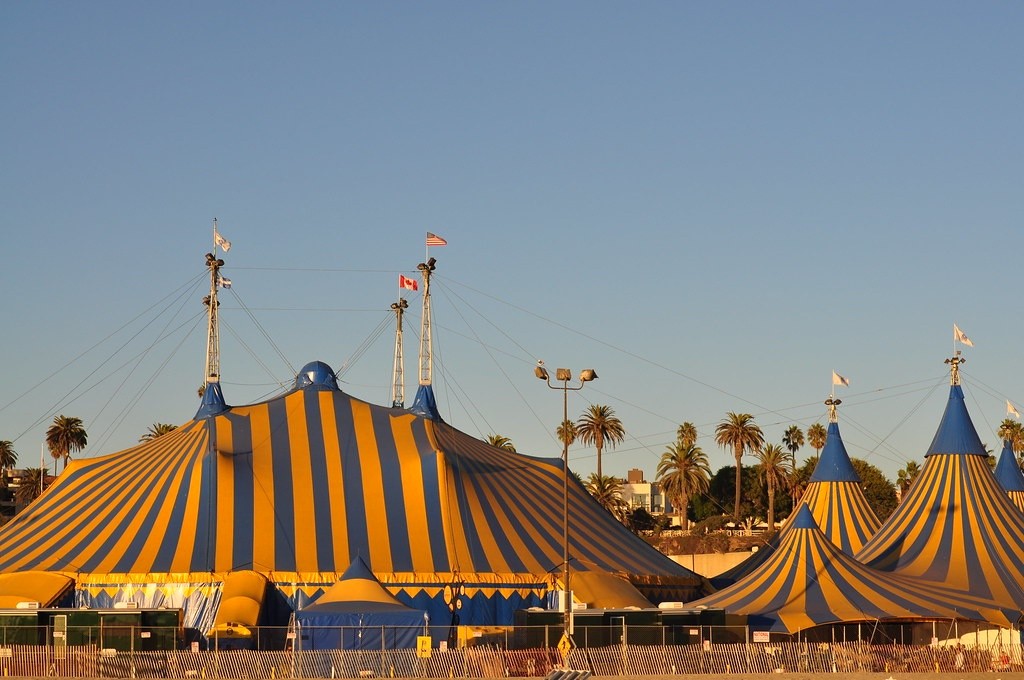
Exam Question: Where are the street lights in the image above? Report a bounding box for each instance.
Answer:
[533,361,599,680]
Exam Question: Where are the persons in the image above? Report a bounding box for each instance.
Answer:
[1,643,992,679]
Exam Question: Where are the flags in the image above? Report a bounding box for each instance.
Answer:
[1007,400,1021,419]
[832,371,850,387]
[215,233,231,253]
[427,232,447,245]
[215,276,232,289]
[954,325,975,347]
[400,275,418,292]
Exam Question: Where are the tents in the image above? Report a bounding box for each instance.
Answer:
[0,361,1024,643]
